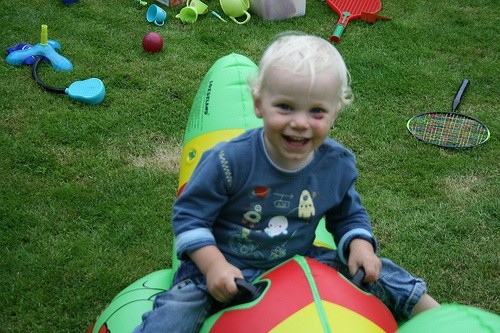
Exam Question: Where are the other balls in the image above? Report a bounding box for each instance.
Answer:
[143,31,162,51]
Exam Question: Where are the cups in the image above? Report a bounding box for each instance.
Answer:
[146,4,167,26]
[219,0,251,24]
[186,0,208,14]
[175,6,198,23]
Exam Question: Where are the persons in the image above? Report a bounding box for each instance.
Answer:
[134,36,441,333]
